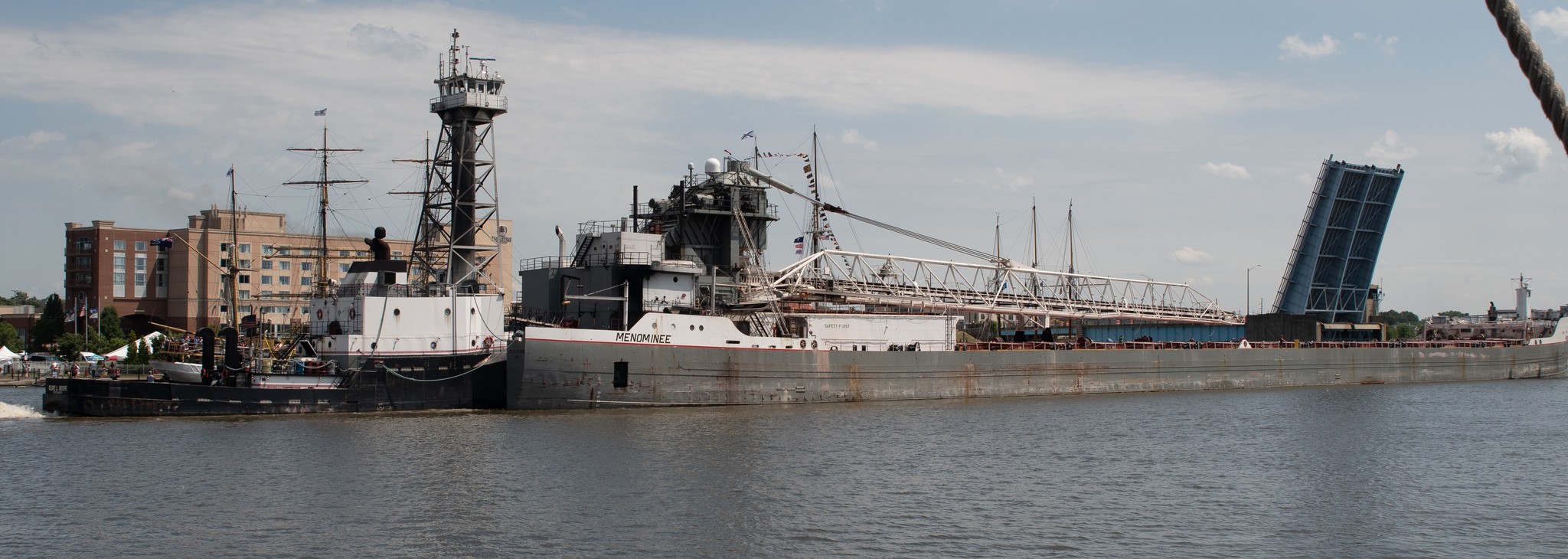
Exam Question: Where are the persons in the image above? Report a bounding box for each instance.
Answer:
[1279,334,1287,349]
[1188,336,1197,350]
[19,334,296,384]
[1118,335,1124,343]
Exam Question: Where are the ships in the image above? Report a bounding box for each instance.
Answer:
[40,31,1567,414]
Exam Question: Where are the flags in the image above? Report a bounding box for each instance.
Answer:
[89,307,98,318]
[738,129,861,282]
[64,307,75,322]
[77,298,86,317]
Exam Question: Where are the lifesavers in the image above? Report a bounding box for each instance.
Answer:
[182,343,189,351]
[317,309,323,319]
[484,335,493,346]
[222,369,228,380]
[349,308,356,319]
[200,368,206,379]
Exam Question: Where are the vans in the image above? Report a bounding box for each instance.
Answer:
[31,355,58,362]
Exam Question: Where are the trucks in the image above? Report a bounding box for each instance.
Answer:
[75,351,98,364]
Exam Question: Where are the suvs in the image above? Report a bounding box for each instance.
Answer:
[21,352,50,361]
